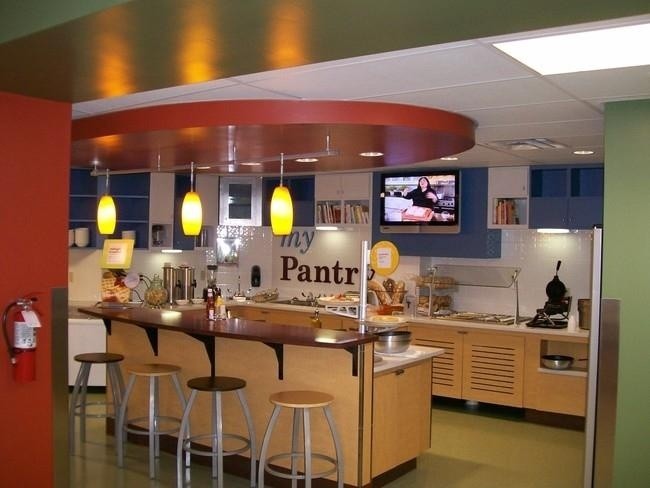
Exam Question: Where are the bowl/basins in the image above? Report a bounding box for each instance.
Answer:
[191,298,204,303]
[175,300,188,305]
[233,295,247,303]
[371,330,411,353]
[74,227,90,247]
[122,230,137,240]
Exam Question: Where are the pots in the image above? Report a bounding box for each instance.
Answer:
[541,354,587,370]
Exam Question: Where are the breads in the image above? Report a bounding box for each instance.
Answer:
[368,278,404,304]
[419,275,452,309]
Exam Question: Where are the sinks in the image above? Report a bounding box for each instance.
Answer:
[226,296,254,301]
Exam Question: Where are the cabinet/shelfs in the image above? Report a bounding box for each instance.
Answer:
[486,162,603,230]
[407,322,524,411]
[69,168,219,253]
[536,335,590,417]
[314,172,372,227]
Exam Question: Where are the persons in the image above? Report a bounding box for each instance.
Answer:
[401,176,441,210]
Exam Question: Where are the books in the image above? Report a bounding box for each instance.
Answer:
[494,198,518,223]
[316,200,369,223]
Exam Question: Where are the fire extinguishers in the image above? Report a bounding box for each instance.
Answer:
[2,291,46,383]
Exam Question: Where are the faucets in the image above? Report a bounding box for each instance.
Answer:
[237,274,241,296]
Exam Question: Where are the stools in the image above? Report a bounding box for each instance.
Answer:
[69,351,346,487]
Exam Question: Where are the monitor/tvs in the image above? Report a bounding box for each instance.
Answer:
[379,169,462,234]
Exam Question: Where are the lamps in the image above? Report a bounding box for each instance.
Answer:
[269,151,295,237]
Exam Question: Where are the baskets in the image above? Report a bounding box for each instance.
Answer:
[254,289,278,303]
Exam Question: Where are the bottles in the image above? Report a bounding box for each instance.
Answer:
[311,309,322,329]
[145,274,169,305]
[206,288,215,320]
[496,198,520,224]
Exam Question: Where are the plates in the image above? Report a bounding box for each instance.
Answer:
[318,296,360,306]
[124,302,142,307]
[363,316,408,328]
